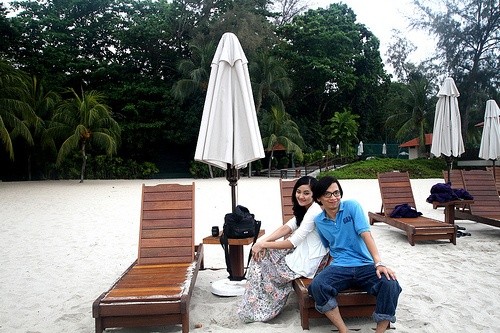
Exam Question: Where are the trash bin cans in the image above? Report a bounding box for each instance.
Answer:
[280,169,288,179]
[295,168,301,178]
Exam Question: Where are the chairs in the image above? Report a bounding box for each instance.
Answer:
[368,171,457,246]
[442,167,500,228]
[280,179,390,331]
[92,182,205,333]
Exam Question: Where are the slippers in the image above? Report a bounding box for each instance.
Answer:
[457,231,471,237]
[457,225,466,230]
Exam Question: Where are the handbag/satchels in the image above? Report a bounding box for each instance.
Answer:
[390,203,422,218]
[224,205,256,239]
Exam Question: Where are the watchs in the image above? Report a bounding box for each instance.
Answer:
[375,261,386,268]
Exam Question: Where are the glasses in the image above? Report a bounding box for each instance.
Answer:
[323,190,340,198]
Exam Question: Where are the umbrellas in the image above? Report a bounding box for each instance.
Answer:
[194,32,265,213]
[336,144,339,155]
[478,100,500,182]
[383,143,386,154]
[357,141,364,155]
[431,77,465,183]
[328,145,331,151]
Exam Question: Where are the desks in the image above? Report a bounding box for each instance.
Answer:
[426,199,474,224]
[203,230,266,277]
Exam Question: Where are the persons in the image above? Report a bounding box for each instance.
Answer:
[238,175,329,324]
[309,176,402,333]
